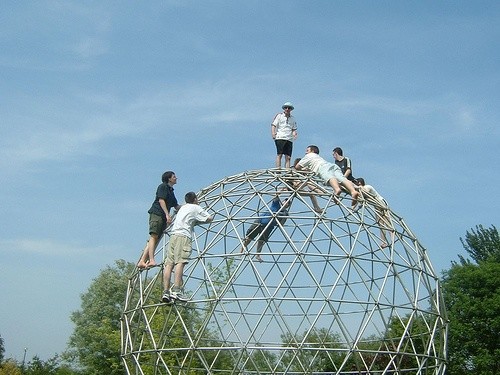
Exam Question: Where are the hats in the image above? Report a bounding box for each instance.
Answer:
[285,197,293,203]
[282,103,294,110]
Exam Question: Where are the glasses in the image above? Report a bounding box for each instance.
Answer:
[283,107,291,110]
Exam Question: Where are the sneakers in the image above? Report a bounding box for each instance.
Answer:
[163,294,174,303]
[170,290,190,301]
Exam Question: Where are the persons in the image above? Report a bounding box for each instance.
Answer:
[137,171,180,267]
[271,102,298,181]
[295,145,359,206]
[355,178,388,248]
[162,192,213,306]
[333,147,359,192]
[285,158,327,214]
[242,187,291,263]
